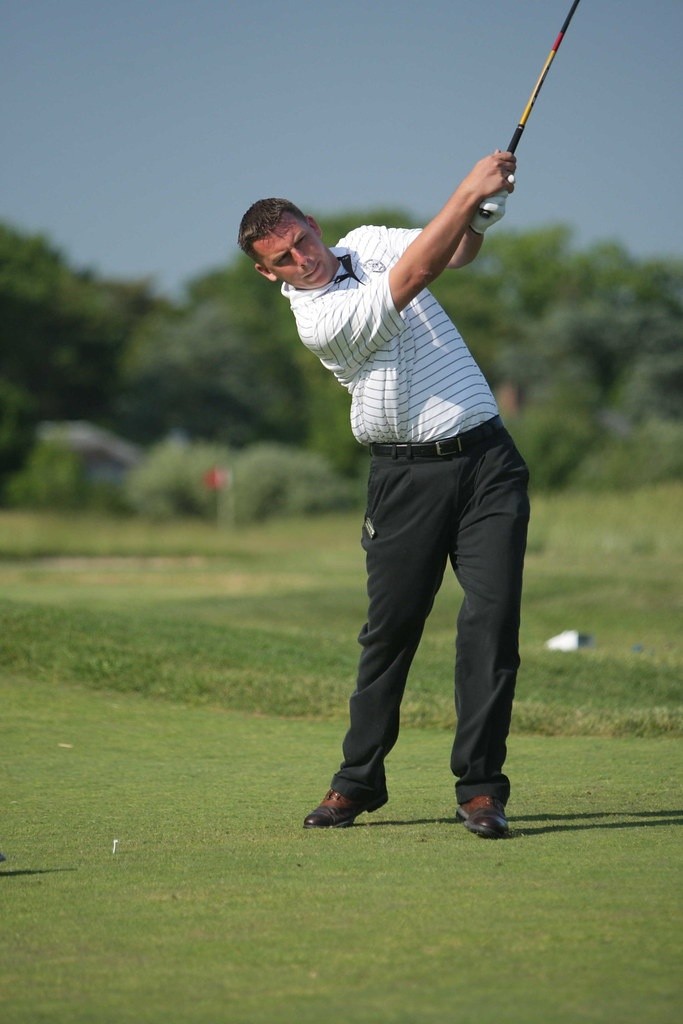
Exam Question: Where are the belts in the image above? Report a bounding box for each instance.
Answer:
[367,415,501,457]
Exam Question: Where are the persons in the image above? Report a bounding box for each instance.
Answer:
[237,148,530,839]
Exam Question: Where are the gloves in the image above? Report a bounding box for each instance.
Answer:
[468,174,517,236]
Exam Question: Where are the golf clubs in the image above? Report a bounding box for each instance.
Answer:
[479,0,580,219]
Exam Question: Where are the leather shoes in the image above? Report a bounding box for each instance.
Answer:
[304,789,389,829]
[456,795,510,839]
